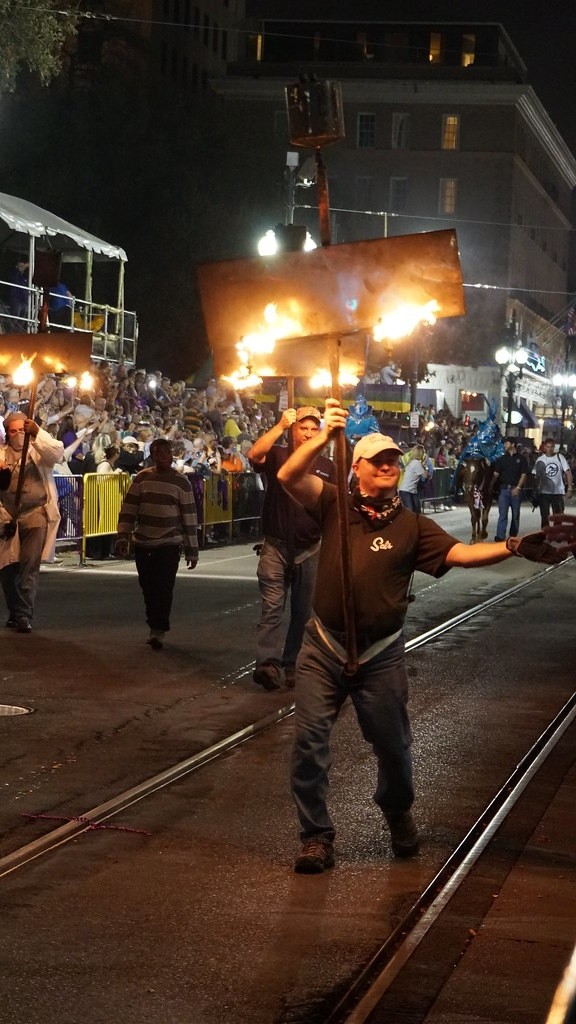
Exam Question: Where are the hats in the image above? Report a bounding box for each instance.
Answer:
[352,432,404,464]
[2,412,28,430]
[501,436,517,445]
[295,407,321,425]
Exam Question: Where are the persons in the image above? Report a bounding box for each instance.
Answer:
[116,438,198,650]
[532,438,573,531]
[487,437,528,542]
[248,406,333,689]
[0,412,64,632]
[0,360,576,559]
[398,444,428,515]
[277,398,569,874]
[6,260,73,334]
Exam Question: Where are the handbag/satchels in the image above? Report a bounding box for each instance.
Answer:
[531,490,539,509]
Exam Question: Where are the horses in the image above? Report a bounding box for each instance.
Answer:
[461,459,494,544]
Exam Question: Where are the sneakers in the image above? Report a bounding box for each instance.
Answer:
[383,811,420,860]
[285,664,297,688]
[255,663,281,691]
[294,840,336,874]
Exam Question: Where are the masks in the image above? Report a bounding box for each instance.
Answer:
[9,432,32,452]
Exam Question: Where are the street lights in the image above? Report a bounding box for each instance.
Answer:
[552,372,576,455]
[493,344,530,437]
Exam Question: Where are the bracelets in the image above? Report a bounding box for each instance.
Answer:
[517,487,521,489]
[568,486,573,491]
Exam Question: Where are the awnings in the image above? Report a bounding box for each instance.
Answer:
[520,399,539,428]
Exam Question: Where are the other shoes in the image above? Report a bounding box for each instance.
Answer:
[6,612,17,628]
[88,522,260,560]
[17,617,32,633]
[145,630,164,652]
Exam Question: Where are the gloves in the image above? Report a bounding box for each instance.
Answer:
[506,532,569,565]
